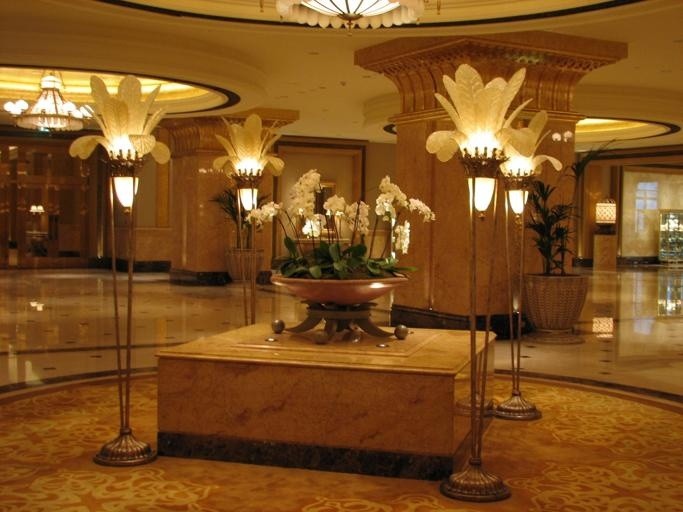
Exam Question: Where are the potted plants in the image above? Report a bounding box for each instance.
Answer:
[209,188,272,290]
[517,138,623,345]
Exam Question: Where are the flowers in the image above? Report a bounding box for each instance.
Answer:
[243,168,436,278]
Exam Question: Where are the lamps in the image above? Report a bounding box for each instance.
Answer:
[4,68,95,133]
[275,0,426,37]
[593,198,617,235]
[69,73,170,467]
[424,62,535,501]
[28,298,45,312]
[592,309,615,340]
[28,204,45,215]
[494,105,563,421]
[211,113,284,331]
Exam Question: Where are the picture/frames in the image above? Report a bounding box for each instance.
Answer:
[312,180,339,235]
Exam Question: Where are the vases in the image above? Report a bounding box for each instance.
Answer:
[267,275,410,344]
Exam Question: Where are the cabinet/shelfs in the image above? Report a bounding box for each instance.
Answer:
[657,209,683,266]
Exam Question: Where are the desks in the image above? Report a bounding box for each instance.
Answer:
[153,322,498,480]
